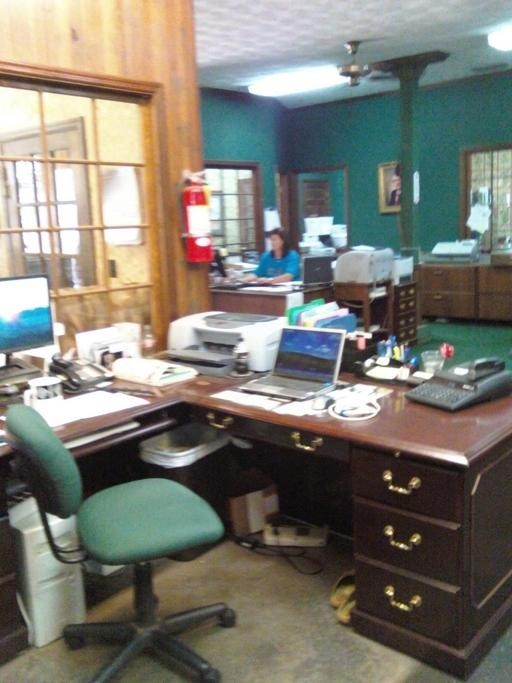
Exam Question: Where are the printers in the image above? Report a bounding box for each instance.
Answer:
[333,245,395,284]
[166,310,290,373]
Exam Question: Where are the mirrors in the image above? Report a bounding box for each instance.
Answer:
[290,165,349,254]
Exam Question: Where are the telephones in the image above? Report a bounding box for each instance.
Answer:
[49,353,114,392]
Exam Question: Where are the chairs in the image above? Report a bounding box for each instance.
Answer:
[1,403,237,682]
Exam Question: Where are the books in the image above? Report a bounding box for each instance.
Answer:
[112,356,198,387]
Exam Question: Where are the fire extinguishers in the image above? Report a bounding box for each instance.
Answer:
[180,171,215,263]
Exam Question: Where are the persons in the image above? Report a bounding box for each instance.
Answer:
[388,175,402,205]
[243,228,300,283]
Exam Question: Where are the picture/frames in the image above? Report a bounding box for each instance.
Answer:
[377,160,402,214]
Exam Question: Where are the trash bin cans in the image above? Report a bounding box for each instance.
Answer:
[136,424,233,561]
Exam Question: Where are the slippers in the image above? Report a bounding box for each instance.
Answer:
[335,589,357,624]
[329,569,358,608]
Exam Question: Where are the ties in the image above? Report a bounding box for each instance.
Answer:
[395,191,400,204]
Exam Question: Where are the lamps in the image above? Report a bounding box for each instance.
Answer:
[338,41,371,87]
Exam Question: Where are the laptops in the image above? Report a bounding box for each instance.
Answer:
[291,255,336,287]
[239,325,347,401]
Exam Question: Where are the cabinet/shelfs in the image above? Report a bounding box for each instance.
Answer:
[391,280,420,349]
[419,261,477,319]
[335,276,391,339]
[477,265,511,320]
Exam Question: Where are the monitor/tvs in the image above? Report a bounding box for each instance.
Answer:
[1,274,56,381]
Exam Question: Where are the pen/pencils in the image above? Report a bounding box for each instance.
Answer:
[393,345,405,361]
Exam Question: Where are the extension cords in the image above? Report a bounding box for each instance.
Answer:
[260,523,330,548]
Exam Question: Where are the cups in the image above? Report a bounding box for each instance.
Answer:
[422,350,445,374]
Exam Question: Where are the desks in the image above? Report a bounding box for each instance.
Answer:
[0,345,512,682]
[210,275,333,314]
